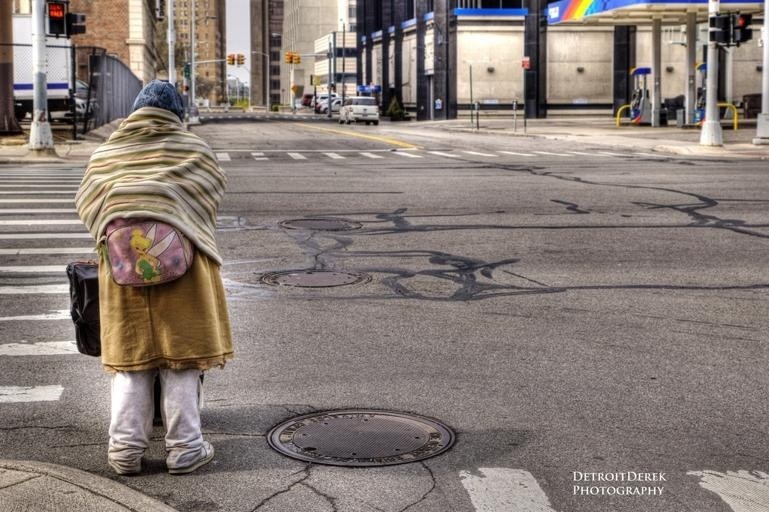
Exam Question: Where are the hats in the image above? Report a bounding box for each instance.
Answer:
[132,80,184,120]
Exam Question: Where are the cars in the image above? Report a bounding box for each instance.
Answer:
[301,93,341,114]
[338,96,378,125]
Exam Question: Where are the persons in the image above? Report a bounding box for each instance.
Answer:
[75,80,235,474]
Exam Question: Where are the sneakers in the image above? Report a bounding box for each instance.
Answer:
[108,456,142,476]
[168,439,215,476]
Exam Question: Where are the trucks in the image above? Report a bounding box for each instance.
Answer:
[11,13,72,122]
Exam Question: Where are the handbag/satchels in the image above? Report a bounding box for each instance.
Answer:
[66,261,101,357]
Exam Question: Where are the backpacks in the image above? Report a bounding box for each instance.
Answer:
[105,218,196,288]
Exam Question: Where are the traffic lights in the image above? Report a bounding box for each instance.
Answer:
[285,51,300,64]
[227,54,234,65]
[44,0,85,39]
[709,12,752,42]
[236,54,244,65]
